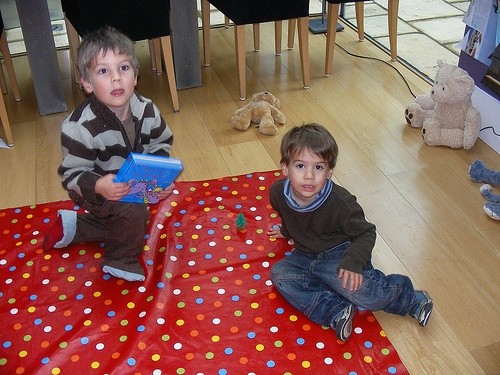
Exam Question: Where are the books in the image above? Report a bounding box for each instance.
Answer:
[109,152,183,203]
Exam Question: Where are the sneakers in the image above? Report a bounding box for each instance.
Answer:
[334,303,356,341]
[408,290,434,327]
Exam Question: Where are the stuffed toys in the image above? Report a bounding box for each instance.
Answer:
[405,61,483,150]
[467,159,500,221]
[231,91,286,136]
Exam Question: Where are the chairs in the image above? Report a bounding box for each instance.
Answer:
[61,0,179,117]
[201,0,311,100]
[323,0,401,78]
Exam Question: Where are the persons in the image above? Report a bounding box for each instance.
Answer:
[266,122,433,341]
[42,29,176,281]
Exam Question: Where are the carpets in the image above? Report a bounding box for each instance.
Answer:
[0,168,410,375]
